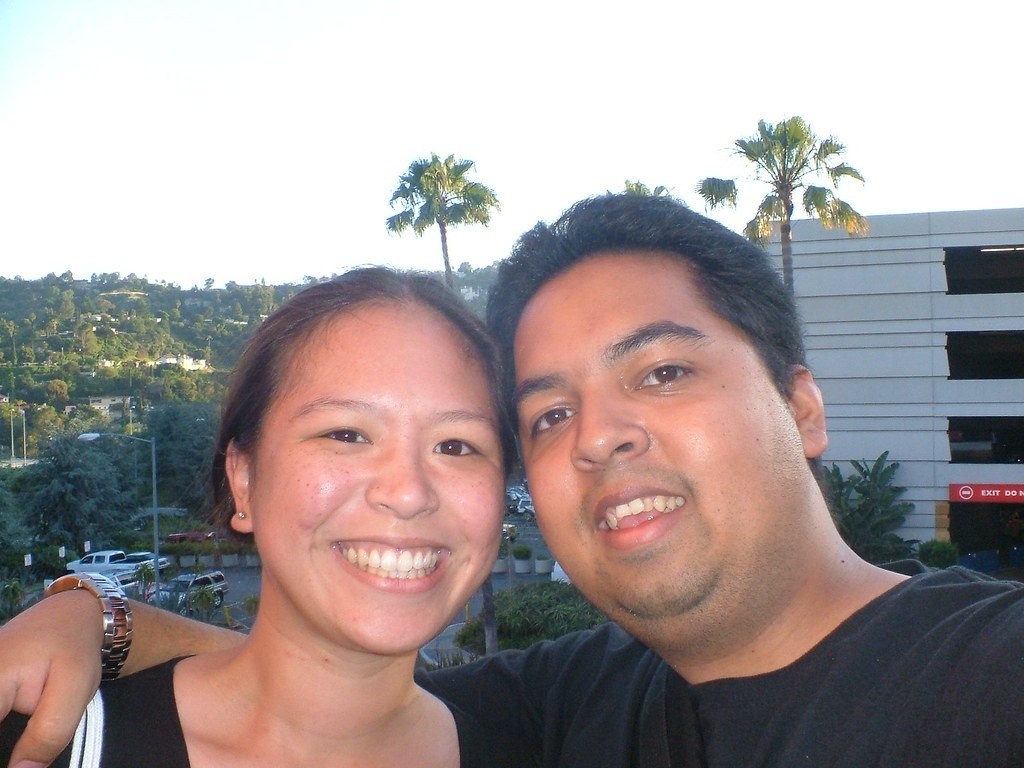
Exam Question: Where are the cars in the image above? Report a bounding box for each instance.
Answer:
[65,547,173,605]
[505,486,537,524]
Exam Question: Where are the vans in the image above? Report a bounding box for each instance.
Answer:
[162,533,221,548]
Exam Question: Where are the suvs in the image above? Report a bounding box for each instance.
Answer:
[147,570,230,618]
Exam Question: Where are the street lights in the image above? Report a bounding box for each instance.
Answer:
[77,431,162,608]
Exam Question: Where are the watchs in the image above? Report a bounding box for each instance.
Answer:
[42,572,134,686]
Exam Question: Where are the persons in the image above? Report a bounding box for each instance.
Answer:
[1,191,1023,768]
[1,263,548,768]
